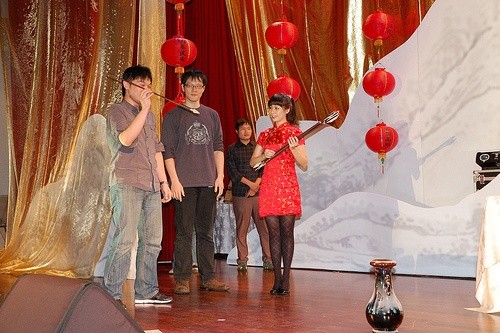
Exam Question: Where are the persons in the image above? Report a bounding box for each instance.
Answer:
[226,120,274,271]
[160,70,230,295]
[106,66,173,309]
[249,93,308,294]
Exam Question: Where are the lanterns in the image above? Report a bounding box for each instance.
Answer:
[361,9,395,60]
[161,35,198,83]
[267,74,301,101]
[365,122,399,174]
[265,19,299,71]
[162,97,186,118]
[165,0,191,19]
[362,68,395,117]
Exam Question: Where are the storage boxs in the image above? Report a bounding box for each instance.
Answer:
[472,170,500,193]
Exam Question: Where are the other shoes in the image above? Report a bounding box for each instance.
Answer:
[264,262,274,269]
[176,280,190,293]
[200,279,230,290]
[238,263,247,271]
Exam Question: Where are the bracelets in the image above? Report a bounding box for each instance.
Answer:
[160,181,168,187]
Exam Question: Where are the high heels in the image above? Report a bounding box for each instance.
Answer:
[270,288,280,295]
[278,288,289,294]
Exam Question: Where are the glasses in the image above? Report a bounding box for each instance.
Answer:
[185,83,204,89]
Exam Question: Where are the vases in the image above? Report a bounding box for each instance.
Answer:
[365,259,404,333]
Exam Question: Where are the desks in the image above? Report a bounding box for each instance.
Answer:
[463,196,500,314]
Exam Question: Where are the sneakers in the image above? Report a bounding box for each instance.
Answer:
[135,293,172,303]
[116,299,126,309]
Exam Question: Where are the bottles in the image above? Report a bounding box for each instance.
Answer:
[365,259,405,333]
